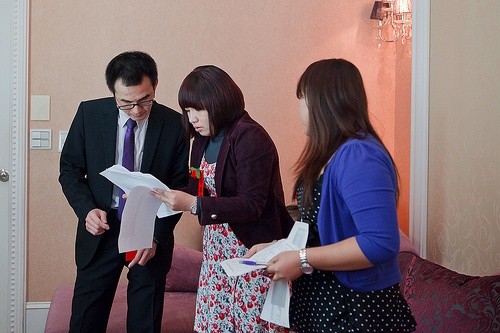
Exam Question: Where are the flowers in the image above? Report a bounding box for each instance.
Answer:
[189,166,203,181]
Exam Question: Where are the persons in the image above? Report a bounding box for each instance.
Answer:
[121,64,295,333]
[57,54,193,333]
[241,58,417,333]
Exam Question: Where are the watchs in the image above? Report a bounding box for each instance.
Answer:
[299,249,314,274]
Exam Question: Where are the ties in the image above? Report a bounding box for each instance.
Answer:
[118,118,137,221]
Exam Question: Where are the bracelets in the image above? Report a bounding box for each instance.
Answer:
[190,196,198,214]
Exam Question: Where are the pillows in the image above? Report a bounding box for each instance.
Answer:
[163,243,203,292]
[400,256,500,333]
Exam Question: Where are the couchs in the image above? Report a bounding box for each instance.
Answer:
[44,204,500,333]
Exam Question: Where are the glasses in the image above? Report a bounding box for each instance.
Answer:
[114,87,156,110]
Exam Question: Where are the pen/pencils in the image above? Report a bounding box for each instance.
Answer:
[240,260,276,265]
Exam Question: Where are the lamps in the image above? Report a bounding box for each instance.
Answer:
[368,0,412,48]
